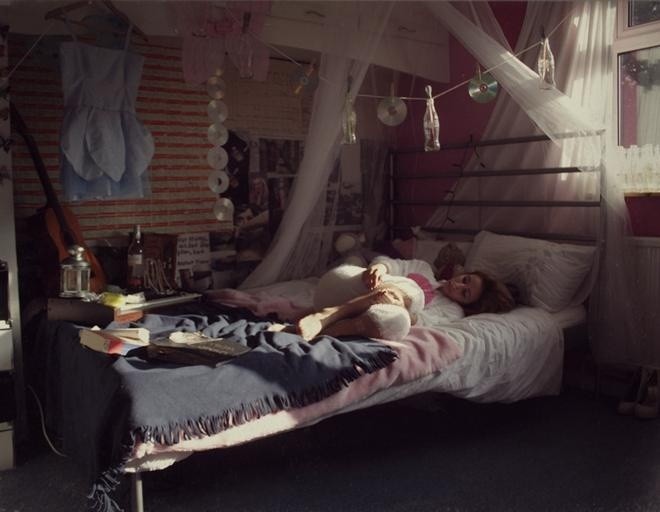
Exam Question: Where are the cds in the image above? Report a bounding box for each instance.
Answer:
[207,170,229,194]
[468,72,498,103]
[376,97,408,126]
[213,198,235,220]
[206,77,225,99]
[208,124,229,146]
[207,146,228,169]
[207,100,229,123]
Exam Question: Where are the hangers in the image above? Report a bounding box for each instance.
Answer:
[44,1,150,45]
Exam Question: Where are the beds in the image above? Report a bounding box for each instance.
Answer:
[26,129,608,510]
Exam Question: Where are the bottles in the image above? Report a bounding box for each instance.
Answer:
[613,143,660,195]
[127,225,145,295]
[238,28,253,80]
[537,36,556,91]
[423,97,441,153]
[341,92,360,146]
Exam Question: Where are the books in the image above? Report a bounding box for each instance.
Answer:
[73,326,252,367]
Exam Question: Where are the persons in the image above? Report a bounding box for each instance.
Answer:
[263,254,524,347]
[365,182,392,229]
[222,139,305,241]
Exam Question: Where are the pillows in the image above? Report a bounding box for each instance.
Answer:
[463,230,600,314]
